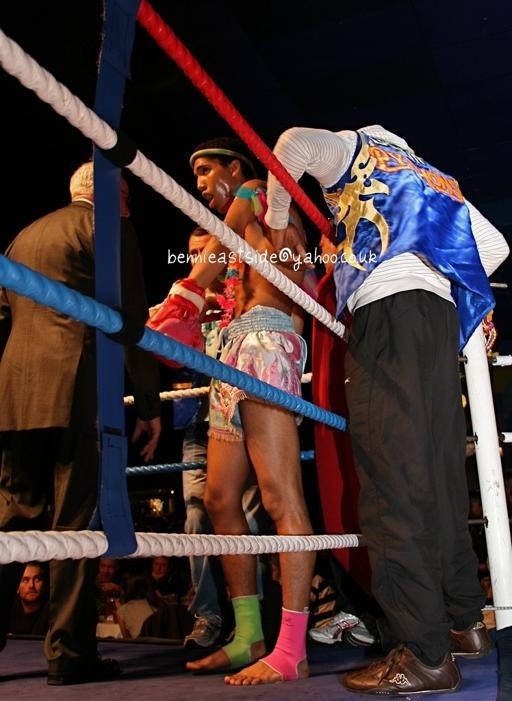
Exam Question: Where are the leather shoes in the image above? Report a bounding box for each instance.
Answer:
[48,658,121,685]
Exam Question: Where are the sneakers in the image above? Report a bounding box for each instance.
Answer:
[184,615,221,648]
[309,611,491,696]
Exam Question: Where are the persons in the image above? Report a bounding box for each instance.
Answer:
[264,122,511,698]
[309,232,401,649]
[143,134,316,687]
[2,481,512,648]
[0,154,149,688]
[171,225,277,650]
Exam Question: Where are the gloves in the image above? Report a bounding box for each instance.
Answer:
[146,278,205,368]
